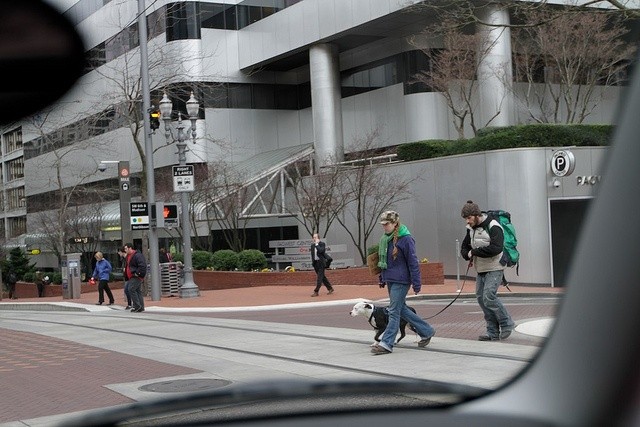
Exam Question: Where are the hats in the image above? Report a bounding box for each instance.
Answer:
[460,200,481,217]
[379,210,399,225]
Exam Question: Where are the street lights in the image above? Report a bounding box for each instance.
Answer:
[160,90,199,299]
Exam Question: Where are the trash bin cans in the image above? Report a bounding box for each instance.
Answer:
[146,263,182,298]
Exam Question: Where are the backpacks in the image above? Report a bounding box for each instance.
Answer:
[467,209,520,292]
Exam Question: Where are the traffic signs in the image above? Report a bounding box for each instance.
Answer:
[172,166,194,193]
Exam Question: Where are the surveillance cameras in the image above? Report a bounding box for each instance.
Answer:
[97,159,108,172]
[553,177,560,188]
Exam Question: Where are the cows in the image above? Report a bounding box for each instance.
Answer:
[349,296,425,349]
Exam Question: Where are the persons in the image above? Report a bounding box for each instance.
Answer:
[461,200,520,341]
[91,251,114,305]
[117,246,132,310]
[370,210,436,355]
[124,243,147,312]
[310,233,335,297]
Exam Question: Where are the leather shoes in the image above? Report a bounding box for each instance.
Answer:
[126,305,131,309]
[96,301,102,305]
[326,288,334,295]
[312,292,318,297]
[138,308,144,312]
[417,328,437,347]
[107,303,114,305]
[479,335,499,341]
[131,308,136,312]
[501,322,517,339]
[372,345,391,354]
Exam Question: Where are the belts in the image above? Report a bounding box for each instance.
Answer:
[314,260,320,262]
